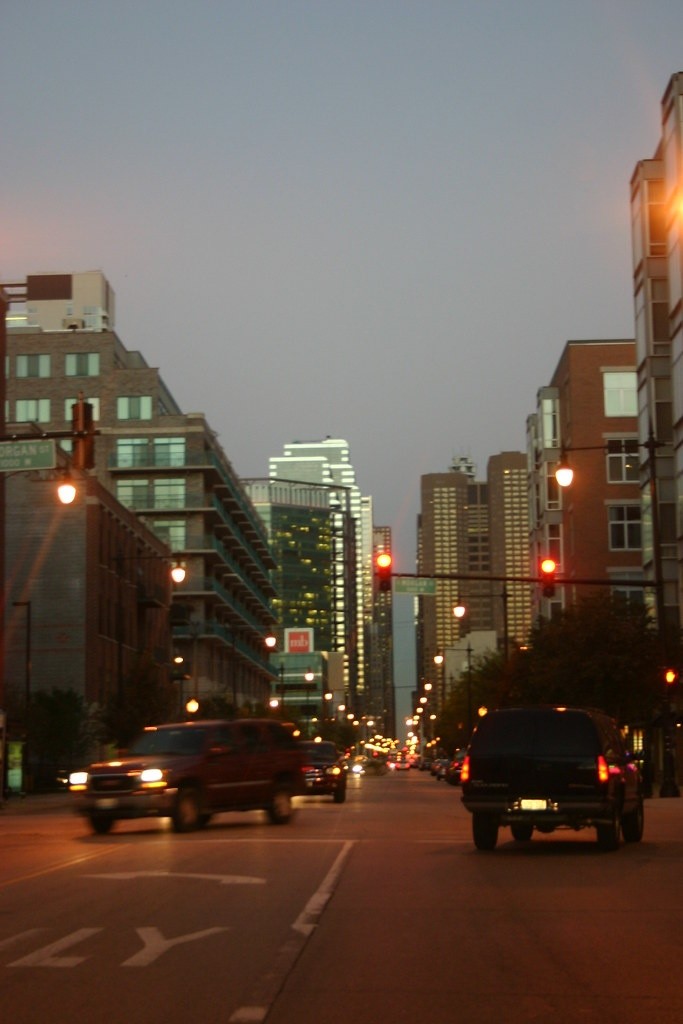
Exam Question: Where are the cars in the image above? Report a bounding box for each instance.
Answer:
[341,741,463,787]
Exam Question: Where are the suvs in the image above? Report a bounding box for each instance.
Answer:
[457,703,647,849]
[296,737,348,804]
[68,717,308,833]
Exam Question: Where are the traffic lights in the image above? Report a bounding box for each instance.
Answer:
[536,555,556,597]
[373,552,393,594]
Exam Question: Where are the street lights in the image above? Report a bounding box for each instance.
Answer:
[108,550,192,711]
[453,589,510,664]
[427,645,476,722]
[280,662,316,715]
[553,435,670,665]
